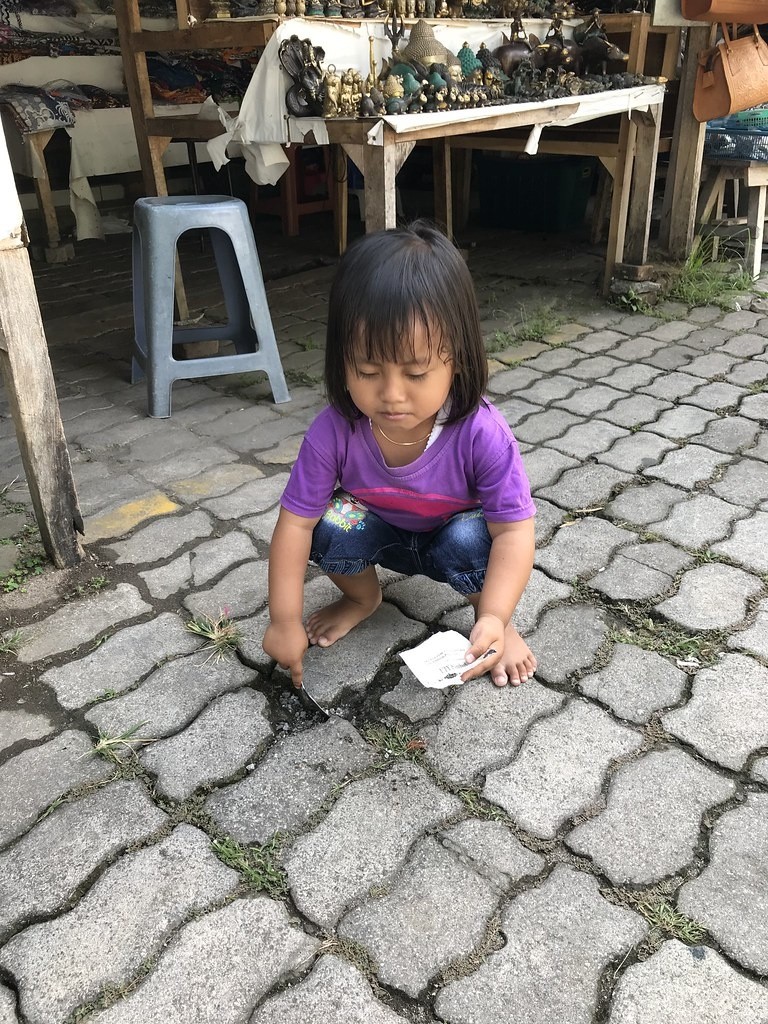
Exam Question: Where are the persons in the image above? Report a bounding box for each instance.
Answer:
[259,219,535,689]
[272,0,644,118]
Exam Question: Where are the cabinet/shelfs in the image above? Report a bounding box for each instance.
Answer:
[114,0,666,297]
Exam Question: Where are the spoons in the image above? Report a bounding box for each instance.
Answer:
[301,681,331,718]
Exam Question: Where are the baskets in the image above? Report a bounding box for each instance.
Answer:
[738,109,768,129]
[703,129,768,160]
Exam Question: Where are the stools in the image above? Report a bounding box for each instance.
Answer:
[249,143,347,256]
[129,196,290,420]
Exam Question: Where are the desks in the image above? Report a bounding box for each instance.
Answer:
[0,12,239,264]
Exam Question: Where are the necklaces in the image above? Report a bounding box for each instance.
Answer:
[378,424,430,446]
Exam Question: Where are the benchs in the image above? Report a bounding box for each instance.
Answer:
[691,158,768,282]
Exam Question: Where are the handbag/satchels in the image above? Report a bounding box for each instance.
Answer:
[692,21,768,123]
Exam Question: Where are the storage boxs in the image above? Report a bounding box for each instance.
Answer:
[473,153,601,231]
